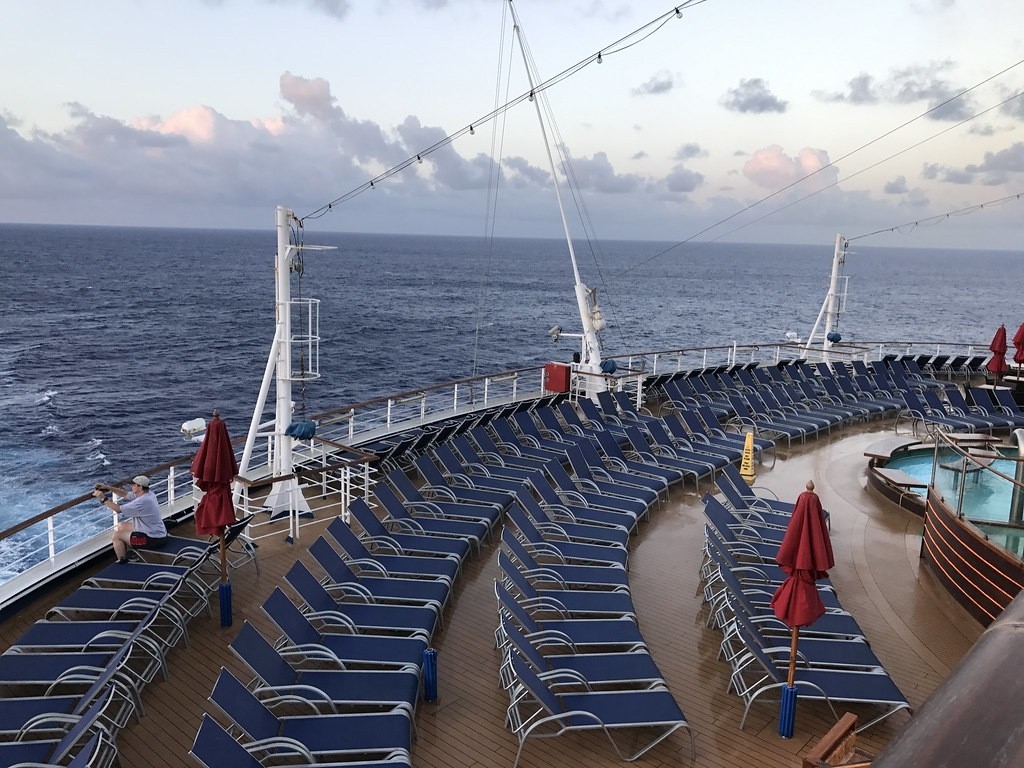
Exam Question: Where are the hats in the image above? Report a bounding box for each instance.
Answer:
[128,475,151,489]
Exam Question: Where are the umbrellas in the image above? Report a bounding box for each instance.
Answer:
[1012,322,1024,381]
[986,324,1008,390]
[192,409,239,583]
[770,479,835,688]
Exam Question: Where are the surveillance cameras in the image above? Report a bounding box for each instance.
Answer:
[549,325,559,334]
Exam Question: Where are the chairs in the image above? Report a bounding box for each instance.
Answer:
[0,354,1024,768]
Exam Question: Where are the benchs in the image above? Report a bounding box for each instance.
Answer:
[864,434,922,458]
[946,433,1003,446]
[940,448,996,490]
[872,466,927,491]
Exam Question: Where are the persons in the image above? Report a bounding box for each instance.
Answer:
[92,476,168,563]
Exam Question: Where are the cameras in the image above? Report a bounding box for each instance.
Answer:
[94,486,102,491]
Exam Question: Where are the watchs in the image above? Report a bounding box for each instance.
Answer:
[101,497,108,503]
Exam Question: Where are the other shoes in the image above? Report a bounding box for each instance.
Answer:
[127,551,139,561]
